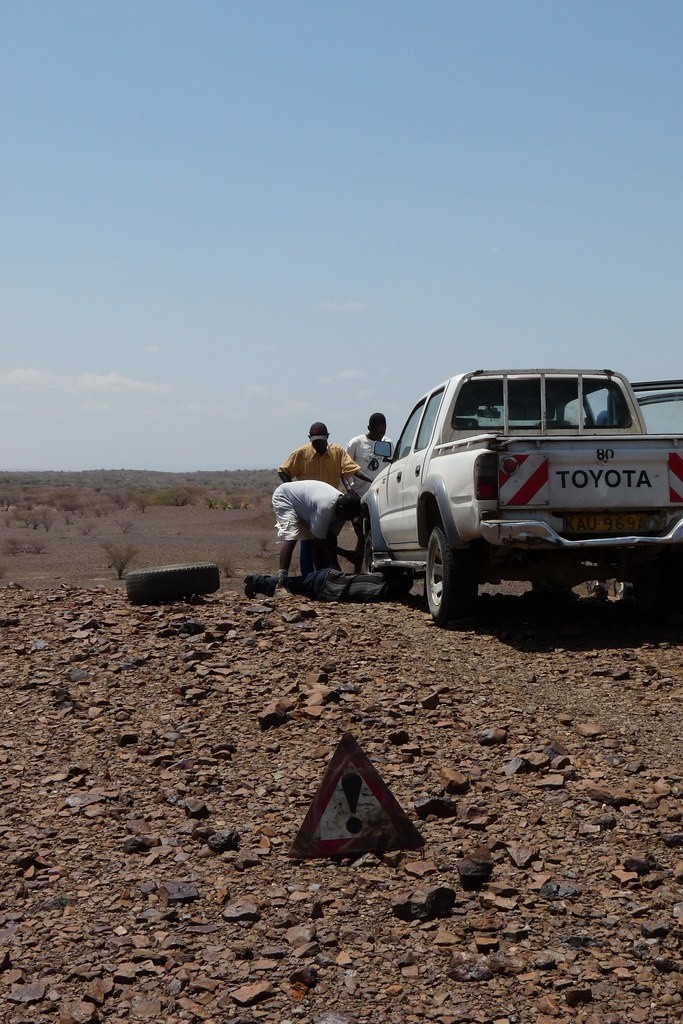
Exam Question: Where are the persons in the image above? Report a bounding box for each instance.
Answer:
[345,412,394,574]
[272,480,361,597]
[278,421,374,572]
[243,546,423,599]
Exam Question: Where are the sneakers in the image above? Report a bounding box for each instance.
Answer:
[244,573,256,599]
[273,583,293,602]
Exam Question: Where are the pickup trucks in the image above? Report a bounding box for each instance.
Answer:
[350,368,682,628]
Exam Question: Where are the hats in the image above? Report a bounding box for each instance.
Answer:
[308,421,327,443]
